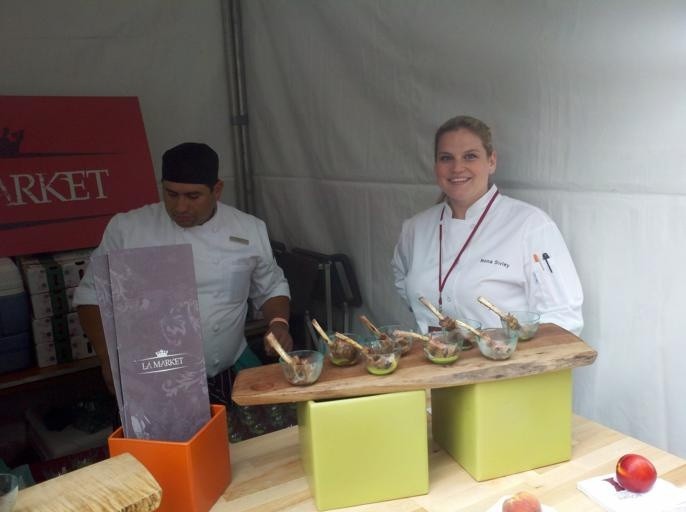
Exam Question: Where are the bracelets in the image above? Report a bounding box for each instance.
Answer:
[269,317,289,327]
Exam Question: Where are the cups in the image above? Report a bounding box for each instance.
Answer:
[442,319,481,351]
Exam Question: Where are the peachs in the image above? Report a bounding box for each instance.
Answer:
[502,490,542,512]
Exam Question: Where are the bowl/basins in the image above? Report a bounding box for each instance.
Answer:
[279,350,324,387]
[500,311,540,342]
[422,331,464,365]
[374,326,413,358]
[475,328,518,360]
[359,340,402,376]
[319,331,364,368]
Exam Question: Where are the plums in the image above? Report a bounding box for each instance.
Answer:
[615,454,657,494]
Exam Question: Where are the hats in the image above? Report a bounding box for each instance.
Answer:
[162,142,220,186]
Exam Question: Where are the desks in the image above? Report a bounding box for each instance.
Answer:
[207,413,686,510]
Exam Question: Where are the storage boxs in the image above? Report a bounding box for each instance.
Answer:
[108,403,232,511]
[296,389,429,512]
[431,369,573,480]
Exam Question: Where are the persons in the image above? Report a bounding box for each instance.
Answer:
[72,142,293,407]
[391,115,584,338]
